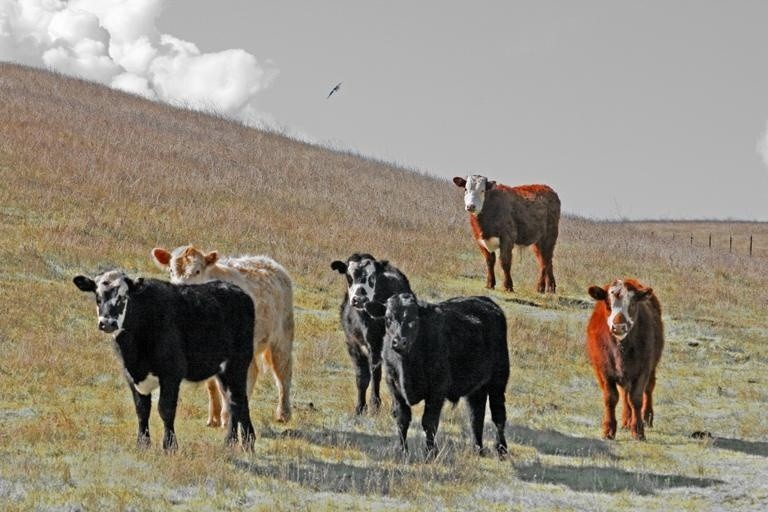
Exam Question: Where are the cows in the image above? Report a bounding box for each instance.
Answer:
[363,293,510,463]
[73,269,255,454]
[586,276,665,443]
[453,175,561,293]
[151,246,294,427]
[330,253,417,417]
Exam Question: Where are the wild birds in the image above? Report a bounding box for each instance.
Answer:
[328,82,342,97]
